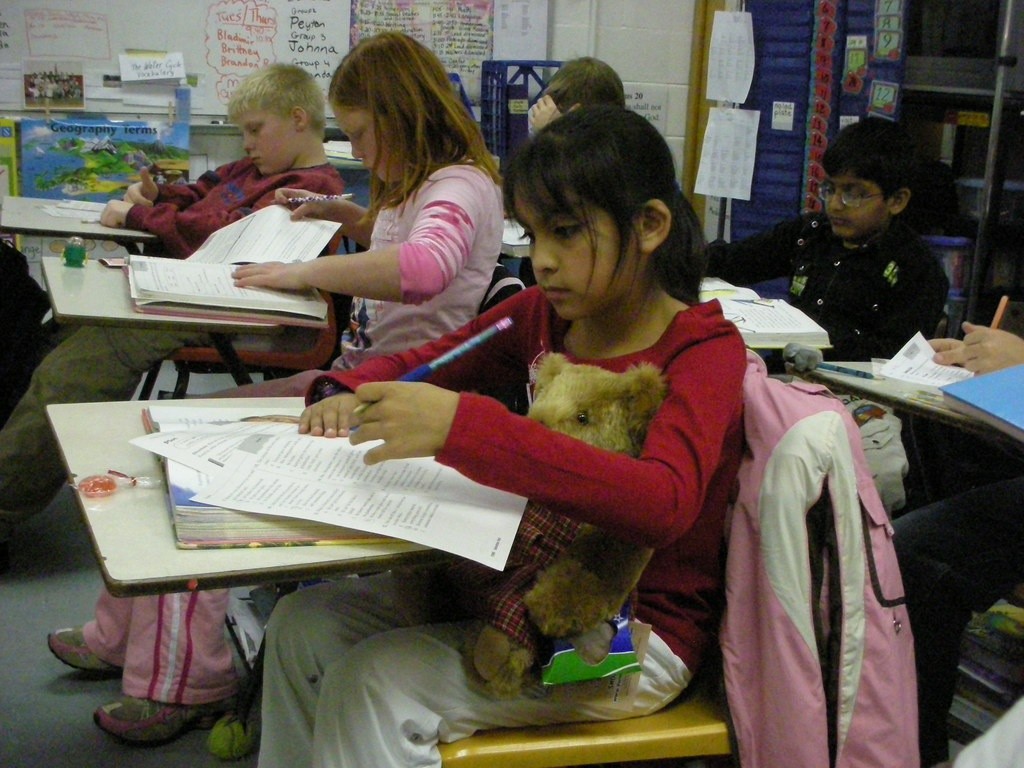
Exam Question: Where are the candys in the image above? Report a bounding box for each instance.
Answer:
[79,476,116,497]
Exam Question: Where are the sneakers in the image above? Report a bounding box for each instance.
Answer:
[48,626,122,672]
[94,693,223,742]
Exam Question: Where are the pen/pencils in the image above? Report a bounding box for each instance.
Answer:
[353,317,515,413]
[990,295,1010,328]
[272,193,354,206]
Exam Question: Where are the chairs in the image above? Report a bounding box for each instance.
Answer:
[437,348,920,768]
[140,224,341,400]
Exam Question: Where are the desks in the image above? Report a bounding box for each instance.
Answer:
[786,361,1013,445]
[42,256,286,387]
[44,397,448,599]
[0,196,157,255]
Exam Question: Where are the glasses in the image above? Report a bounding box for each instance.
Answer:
[818,180,884,209]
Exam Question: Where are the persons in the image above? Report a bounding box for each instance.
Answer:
[249,107,746,768]
[682,117,949,395]
[514,56,625,140]
[39,33,504,745]
[0,61,341,578]
[859,319,1024,768]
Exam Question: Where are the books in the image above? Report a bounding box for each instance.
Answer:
[879,326,1024,444]
[496,212,532,245]
[318,127,364,164]
[51,188,103,222]
[126,203,342,320]
[699,262,829,350]
[693,106,766,205]
[686,8,763,101]
[140,392,531,572]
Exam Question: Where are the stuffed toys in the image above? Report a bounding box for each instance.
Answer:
[463,341,666,701]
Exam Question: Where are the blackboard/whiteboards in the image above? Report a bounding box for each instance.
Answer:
[0,0,557,124]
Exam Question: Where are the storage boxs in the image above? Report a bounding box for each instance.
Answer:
[482,60,562,172]
[958,178,1024,231]
[921,235,1018,305]
[0,87,191,254]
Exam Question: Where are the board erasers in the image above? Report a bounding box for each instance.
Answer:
[210,119,220,125]
